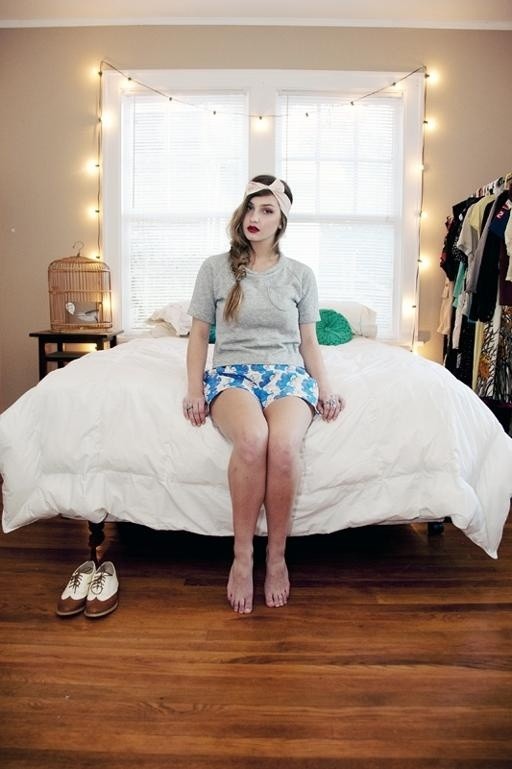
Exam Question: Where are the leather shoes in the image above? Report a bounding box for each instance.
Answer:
[55,561,119,617]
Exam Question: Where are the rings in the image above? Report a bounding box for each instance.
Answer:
[186,404,192,412]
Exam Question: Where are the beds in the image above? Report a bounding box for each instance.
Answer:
[0,329,512,568]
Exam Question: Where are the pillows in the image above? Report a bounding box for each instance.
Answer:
[145,298,379,340]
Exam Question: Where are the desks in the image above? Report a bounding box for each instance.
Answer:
[29,329,125,381]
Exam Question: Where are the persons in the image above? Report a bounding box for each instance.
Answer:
[181,174,345,614]
